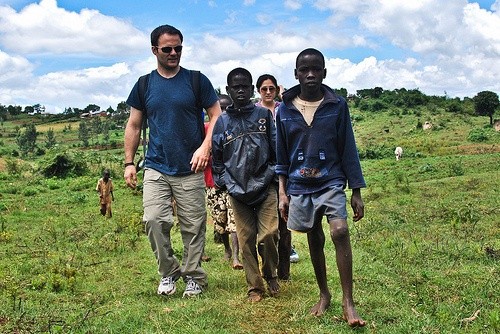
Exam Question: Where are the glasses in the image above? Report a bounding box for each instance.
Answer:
[155,45,182,53]
[259,86,277,93]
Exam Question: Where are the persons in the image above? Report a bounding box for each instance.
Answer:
[96,169,114,218]
[202,94,244,270]
[124,24,223,300]
[250,74,300,279]
[211,68,281,304]
[276,47,367,328]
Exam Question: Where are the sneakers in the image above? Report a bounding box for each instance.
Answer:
[157,273,180,296]
[290,245,300,262]
[182,275,202,298]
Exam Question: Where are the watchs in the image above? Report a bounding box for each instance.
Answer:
[124,162,135,168]
